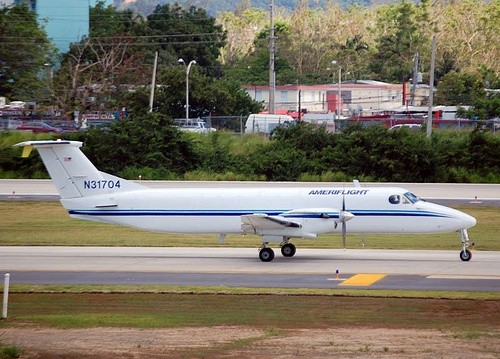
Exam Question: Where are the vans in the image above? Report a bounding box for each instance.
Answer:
[387,124,423,131]
[245,114,297,134]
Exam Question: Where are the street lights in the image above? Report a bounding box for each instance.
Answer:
[44,63,56,107]
[177,58,197,123]
[326,60,345,122]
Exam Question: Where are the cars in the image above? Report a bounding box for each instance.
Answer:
[16,122,63,133]
[177,122,216,134]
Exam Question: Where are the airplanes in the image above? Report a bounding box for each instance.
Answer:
[13,139,479,263]
